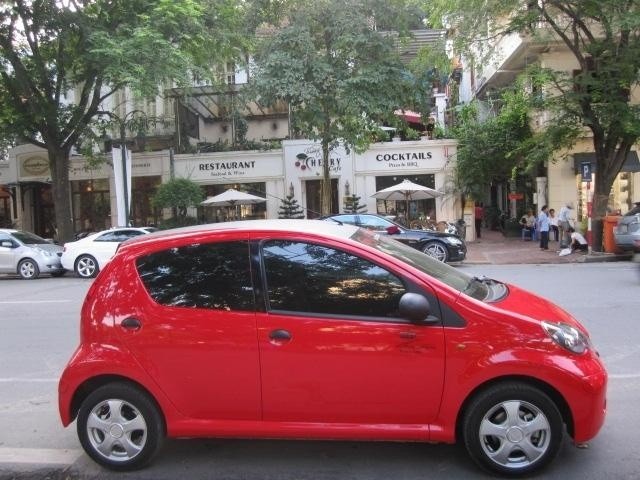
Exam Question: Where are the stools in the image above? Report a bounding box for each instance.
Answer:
[522,227,537,242]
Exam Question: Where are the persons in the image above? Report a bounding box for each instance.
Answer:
[547,209,559,242]
[557,201,576,245]
[475,200,484,238]
[537,203,550,252]
[518,207,536,234]
[565,227,588,252]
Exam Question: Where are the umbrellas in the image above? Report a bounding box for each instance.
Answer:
[369,178,447,228]
[199,187,270,220]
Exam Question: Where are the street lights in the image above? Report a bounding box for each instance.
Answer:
[97,110,148,229]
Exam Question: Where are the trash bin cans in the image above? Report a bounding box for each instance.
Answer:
[602,216,624,254]
[484,206,499,231]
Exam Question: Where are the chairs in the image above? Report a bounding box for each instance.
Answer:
[410,218,449,233]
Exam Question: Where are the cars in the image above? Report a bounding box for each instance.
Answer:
[0,227,166,279]
[318,214,466,263]
[59,219,607,480]
[613,201,640,251]
